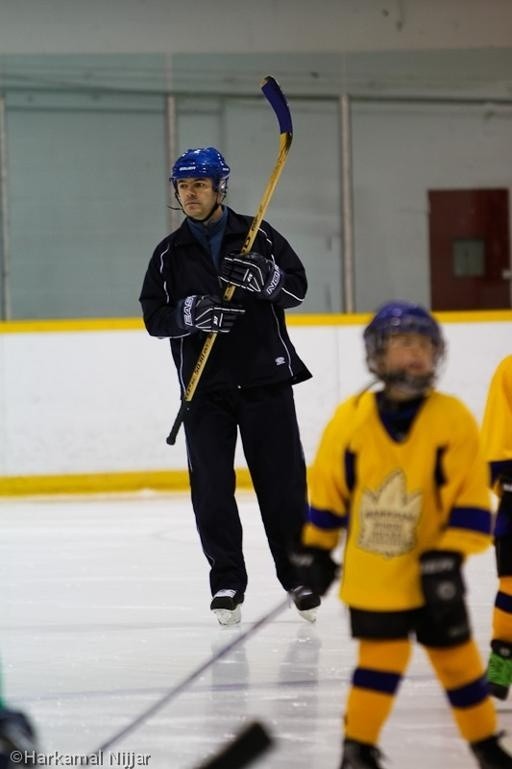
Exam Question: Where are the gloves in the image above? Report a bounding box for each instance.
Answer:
[216,251,286,300]
[419,549,465,607]
[183,294,246,333]
[289,546,341,594]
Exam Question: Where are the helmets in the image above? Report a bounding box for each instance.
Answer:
[170,146,230,191]
[362,302,445,393]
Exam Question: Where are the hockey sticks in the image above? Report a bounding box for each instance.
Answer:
[166,74,291,443]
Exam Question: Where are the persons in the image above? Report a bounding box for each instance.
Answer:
[295,300,512,769]
[139,145,322,612]
[479,354,512,700]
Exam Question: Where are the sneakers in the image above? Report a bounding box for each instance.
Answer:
[292,585,321,610]
[210,588,244,610]
[486,640,512,699]
[341,738,382,769]
[470,734,512,769]
